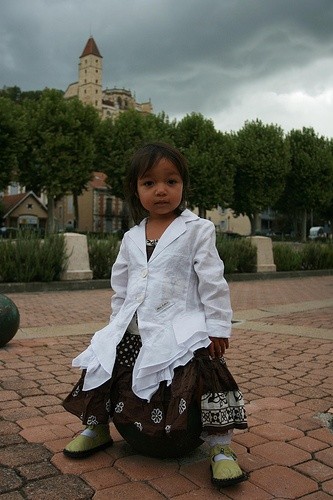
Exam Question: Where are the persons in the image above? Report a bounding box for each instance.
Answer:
[62,141,243,486]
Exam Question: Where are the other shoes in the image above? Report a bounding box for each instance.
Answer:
[63,425,113,458]
[208,445,245,487]
